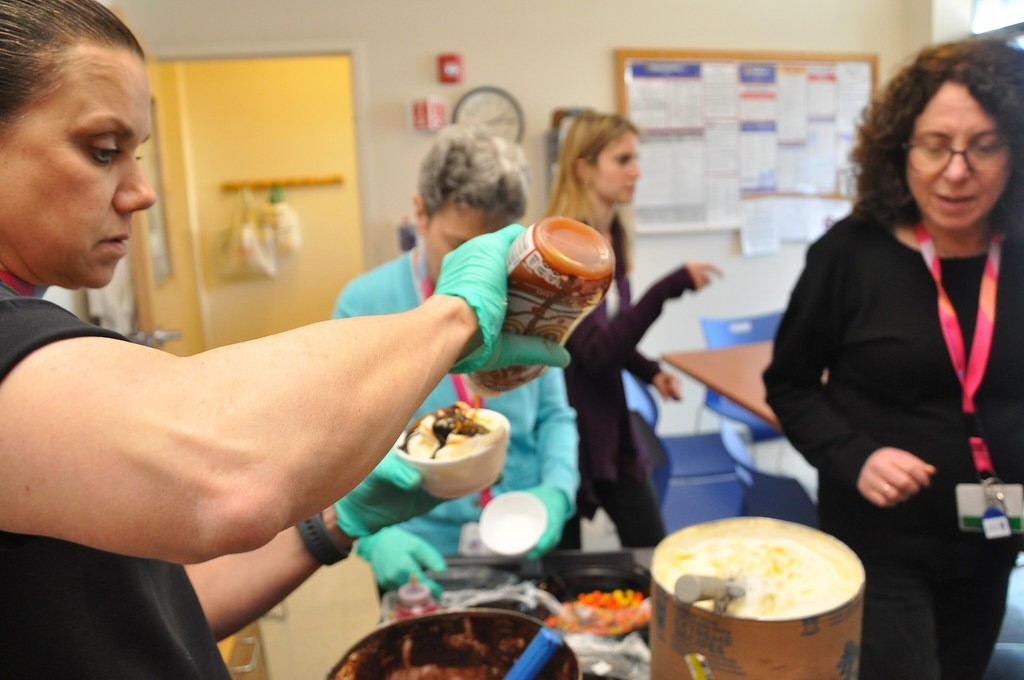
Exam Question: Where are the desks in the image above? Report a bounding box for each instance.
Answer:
[664,339,780,433]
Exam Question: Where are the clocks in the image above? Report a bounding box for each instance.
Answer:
[451,86,527,144]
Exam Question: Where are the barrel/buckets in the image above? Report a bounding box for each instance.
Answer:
[649,516,867,680]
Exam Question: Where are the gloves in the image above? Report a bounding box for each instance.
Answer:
[522,484,569,561]
[353,524,446,601]
[432,224,572,374]
[332,444,503,537]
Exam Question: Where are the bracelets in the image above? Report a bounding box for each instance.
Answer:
[297,512,352,565]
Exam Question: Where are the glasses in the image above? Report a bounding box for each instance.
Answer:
[900,139,1017,181]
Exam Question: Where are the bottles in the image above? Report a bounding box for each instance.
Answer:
[394,572,440,617]
[460,213,616,400]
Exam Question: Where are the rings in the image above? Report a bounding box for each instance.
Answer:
[880,483,891,496]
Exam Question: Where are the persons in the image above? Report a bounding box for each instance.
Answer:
[332,114,723,619]
[0,1,572,680]
[762,41,1024,680]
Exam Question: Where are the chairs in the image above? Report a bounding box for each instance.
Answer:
[702,312,787,515]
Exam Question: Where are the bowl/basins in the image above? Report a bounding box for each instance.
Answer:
[390,406,512,499]
[327,606,584,680]
[478,491,548,557]
